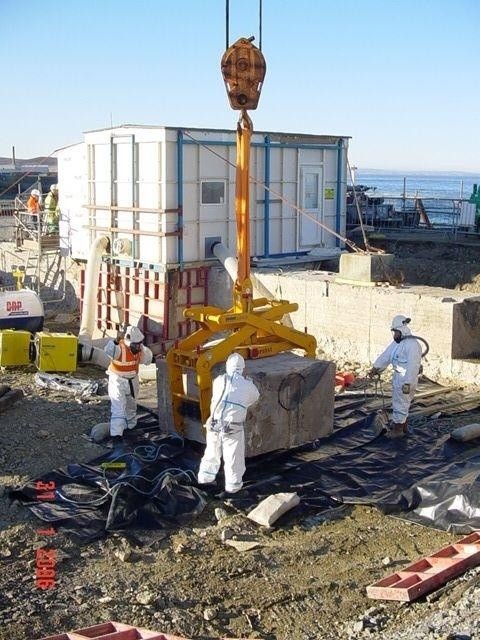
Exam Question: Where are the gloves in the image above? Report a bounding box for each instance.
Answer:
[369,368,379,378]
[401,383,410,393]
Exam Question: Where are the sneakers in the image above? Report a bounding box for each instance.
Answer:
[226,489,249,499]
[198,481,217,489]
[112,428,143,444]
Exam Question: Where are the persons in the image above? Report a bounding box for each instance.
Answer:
[368,314,423,439]
[197,351,262,494]
[55,183,59,217]
[104,324,153,441]
[24,188,44,239]
[42,183,58,238]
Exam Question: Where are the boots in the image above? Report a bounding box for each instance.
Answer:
[385,422,408,438]
